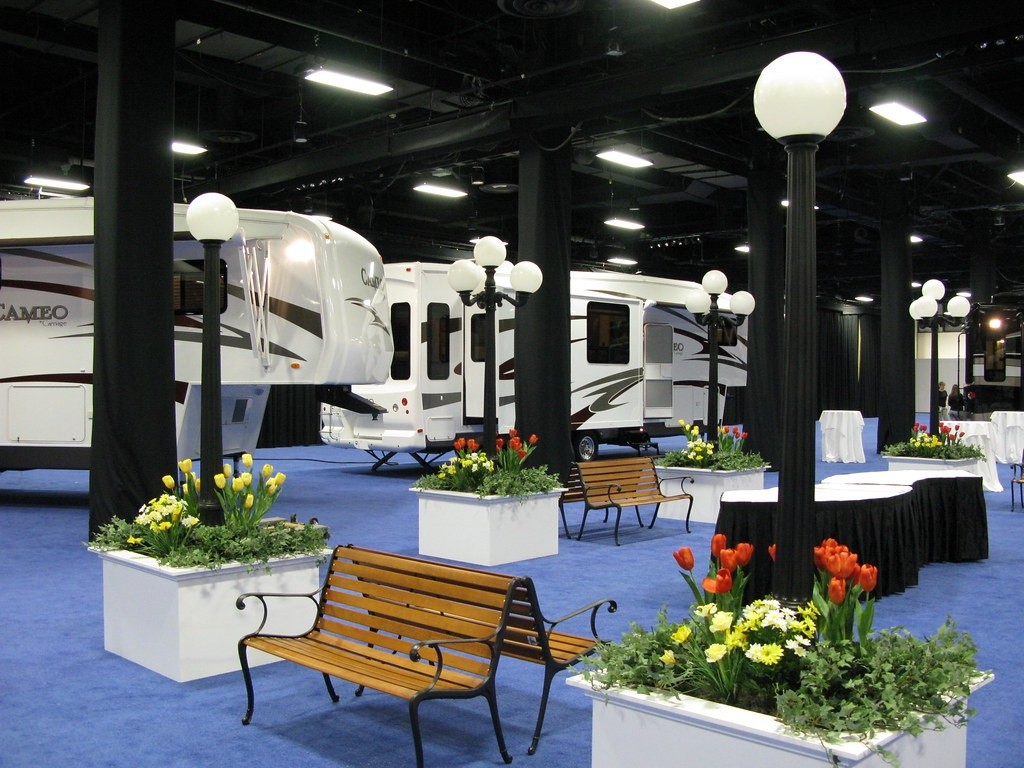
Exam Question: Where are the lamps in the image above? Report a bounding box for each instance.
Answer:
[470,157,484,184]
[897,145,914,182]
[293,77,309,144]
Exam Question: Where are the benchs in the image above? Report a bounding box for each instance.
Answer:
[1011,450,1024,512]
[234,544,618,768]
[557,456,694,546]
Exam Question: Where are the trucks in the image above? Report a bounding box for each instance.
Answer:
[314,259,749,465]
[0,197,395,479]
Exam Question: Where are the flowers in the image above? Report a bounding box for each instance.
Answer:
[649,417,773,474]
[561,531,991,767]
[407,425,564,507]
[88,451,329,574]
[878,421,985,463]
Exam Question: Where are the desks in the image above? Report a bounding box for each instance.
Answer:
[711,465,989,604]
[989,411,1024,465]
[937,420,1003,492]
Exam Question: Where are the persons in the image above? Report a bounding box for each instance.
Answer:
[938,381,963,421]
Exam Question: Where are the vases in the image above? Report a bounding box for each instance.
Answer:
[564,668,995,767]
[409,486,568,567]
[882,455,980,474]
[655,466,772,524]
[89,537,334,683]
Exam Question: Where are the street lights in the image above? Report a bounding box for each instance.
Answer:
[685,270,755,454]
[447,236,542,465]
[186,191,240,526]
[753,50,847,615]
[909,279,971,438]
